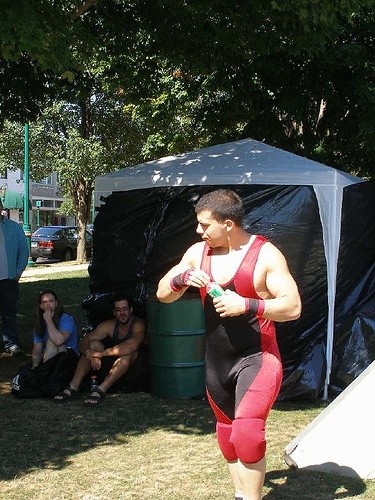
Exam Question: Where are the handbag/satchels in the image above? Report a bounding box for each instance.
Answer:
[12,350,77,398]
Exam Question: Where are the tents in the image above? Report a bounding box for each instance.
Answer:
[85,137,375,401]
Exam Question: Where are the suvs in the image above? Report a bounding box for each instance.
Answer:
[30,226,93,262]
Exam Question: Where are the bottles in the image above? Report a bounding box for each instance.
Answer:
[90,376,99,393]
[205,282,224,303]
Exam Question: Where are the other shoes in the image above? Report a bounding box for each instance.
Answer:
[7,345,21,353]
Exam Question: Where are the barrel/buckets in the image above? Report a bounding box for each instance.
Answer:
[144,292,206,402]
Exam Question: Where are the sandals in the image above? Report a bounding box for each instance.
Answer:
[53,386,78,401]
[83,386,106,408]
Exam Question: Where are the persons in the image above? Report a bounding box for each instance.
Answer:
[54,293,147,405]
[157,188,302,500]
[0,199,28,354]
[31,288,80,369]
